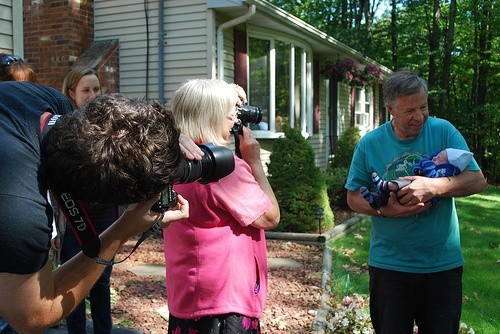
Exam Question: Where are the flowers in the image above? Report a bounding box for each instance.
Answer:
[324,57,383,89]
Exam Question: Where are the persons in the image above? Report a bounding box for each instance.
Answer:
[345,72,486,334]
[0,53,204,334]
[161,78,281,334]
[360,148,473,211]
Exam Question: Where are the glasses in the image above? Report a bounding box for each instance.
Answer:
[0,56,24,66]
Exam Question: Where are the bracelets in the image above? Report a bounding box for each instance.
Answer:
[376,208,385,217]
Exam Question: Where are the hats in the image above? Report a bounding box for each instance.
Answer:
[446,147,474,172]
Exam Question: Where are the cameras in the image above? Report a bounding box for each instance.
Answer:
[151,142,236,213]
[230,101,263,135]
